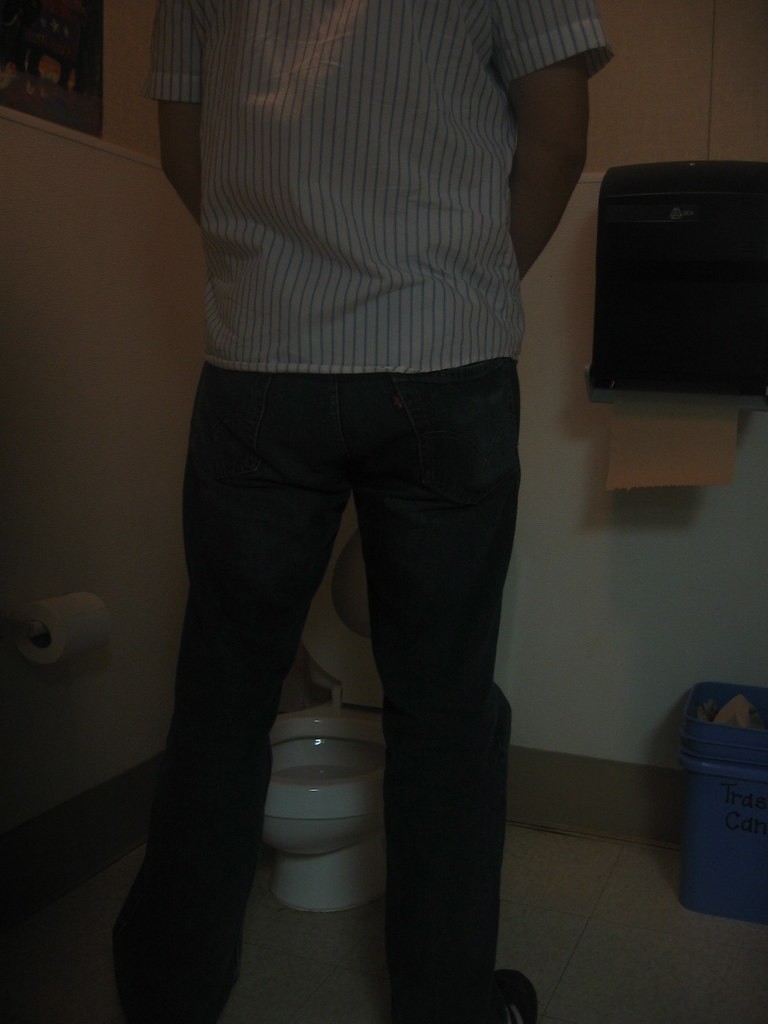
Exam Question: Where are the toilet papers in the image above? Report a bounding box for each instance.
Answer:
[13,590,113,666]
[604,387,742,492]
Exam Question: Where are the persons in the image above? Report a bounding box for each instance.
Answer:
[111,2,612,1023]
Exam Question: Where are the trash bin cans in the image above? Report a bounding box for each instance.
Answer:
[675,681,768,925]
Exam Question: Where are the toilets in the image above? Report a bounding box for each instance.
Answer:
[255,493,389,911]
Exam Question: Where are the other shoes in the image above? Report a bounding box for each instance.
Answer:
[490,969,537,1024]
